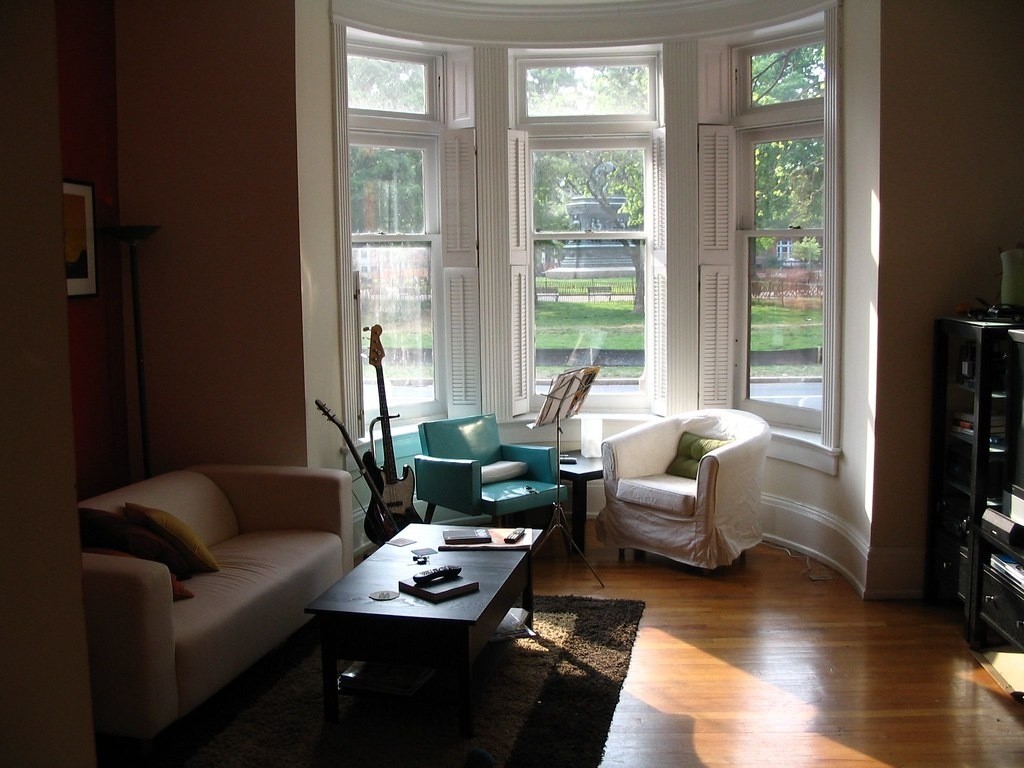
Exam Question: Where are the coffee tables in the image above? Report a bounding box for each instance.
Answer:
[305,523,544,738]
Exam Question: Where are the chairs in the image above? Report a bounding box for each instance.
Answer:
[595,409,770,577]
[414,412,568,538]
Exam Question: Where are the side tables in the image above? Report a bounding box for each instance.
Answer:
[560,450,603,551]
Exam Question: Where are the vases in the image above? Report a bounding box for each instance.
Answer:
[1000,249,1024,308]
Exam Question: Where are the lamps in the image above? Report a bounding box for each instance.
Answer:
[98,224,163,479]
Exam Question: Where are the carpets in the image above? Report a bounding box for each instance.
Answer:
[95,593,646,768]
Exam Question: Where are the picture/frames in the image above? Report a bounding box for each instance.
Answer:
[62,178,99,298]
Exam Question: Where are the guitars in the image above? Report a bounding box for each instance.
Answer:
[315,399,400,532]
[360,324,424,547]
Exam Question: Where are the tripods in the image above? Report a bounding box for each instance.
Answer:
[530,373,605,588]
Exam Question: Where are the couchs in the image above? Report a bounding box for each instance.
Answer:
[79,464,354,757]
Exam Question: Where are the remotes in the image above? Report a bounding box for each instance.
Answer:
[504,528,526,543]
[413,565,462,585]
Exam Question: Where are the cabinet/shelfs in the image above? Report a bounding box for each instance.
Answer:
[924,318,1024,653]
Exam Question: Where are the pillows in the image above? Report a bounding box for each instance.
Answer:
[84,548,195,600]
[481,461,527,483]
[665,432,733,480]
[125,502,220,572]
[79,507,192,579]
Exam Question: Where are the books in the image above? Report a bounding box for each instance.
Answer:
[951,411,1005,439]
[443,528,491,543]
